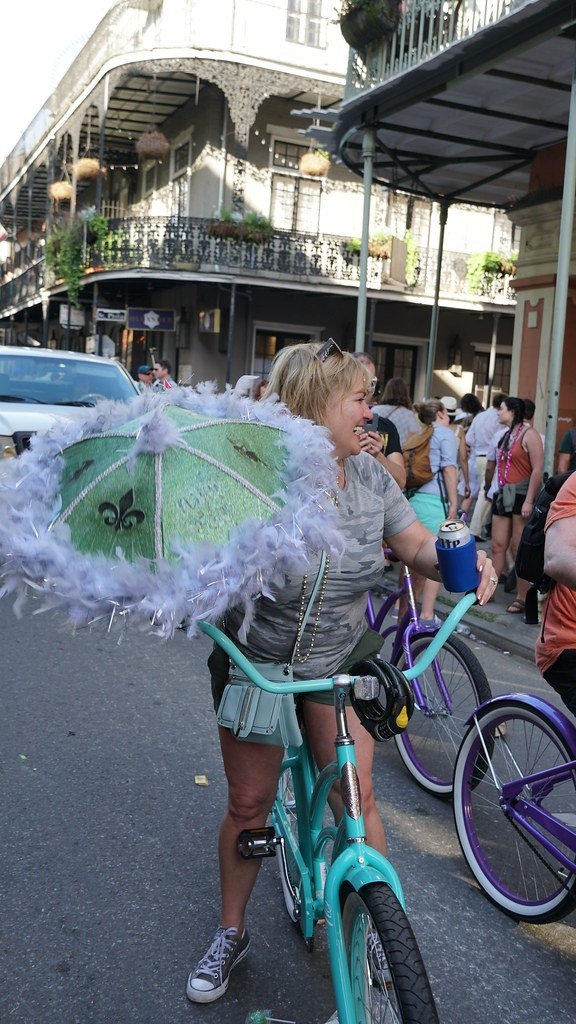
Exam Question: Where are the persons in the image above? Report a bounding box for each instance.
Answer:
[534,471,576,714]
[367,376,576,611]
[187,342,499,1004]
[353,350,404,489]
[138,360,179,387]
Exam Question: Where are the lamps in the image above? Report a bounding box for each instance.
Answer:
[478,313,484,320]
[447,344,463,377]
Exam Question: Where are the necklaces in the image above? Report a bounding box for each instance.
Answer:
[296,465,342,663]
[499,421,523,485]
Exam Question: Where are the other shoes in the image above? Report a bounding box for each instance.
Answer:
[472,534,487,543]
[505,564,518,592]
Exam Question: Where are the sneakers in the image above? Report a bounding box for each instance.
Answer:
[187,926,251,1003]
[366,929,392,983]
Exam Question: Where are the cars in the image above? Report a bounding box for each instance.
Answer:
[0,346,145,463]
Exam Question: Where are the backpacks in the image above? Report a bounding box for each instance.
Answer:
[402,425,442,489]
[514,470,574,624]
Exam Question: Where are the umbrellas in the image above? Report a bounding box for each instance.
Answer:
[0,376,344,637]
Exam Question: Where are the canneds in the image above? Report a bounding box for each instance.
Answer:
[437,519,470,548]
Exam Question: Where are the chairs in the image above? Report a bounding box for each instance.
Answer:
[73,374,127,404]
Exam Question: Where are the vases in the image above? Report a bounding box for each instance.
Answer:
[170,254,200,272]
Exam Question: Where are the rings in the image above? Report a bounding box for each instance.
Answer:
[490,578,497,585]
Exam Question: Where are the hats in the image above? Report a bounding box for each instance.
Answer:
[138,365,153,374]
[441,396,457,416]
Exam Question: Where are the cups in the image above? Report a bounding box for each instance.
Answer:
[435,534,479,592]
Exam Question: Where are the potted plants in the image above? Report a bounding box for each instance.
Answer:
[73,157,108,183]
[465,249,519,297]
[298,147,331,177]
[48,180,79,200]
[135,130,170,162]
[207,206,275,245]
[40,211,141,311]
[329,0,401,50]
[347,227,418,289]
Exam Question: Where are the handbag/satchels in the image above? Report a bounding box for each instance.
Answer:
[215,661,303,749]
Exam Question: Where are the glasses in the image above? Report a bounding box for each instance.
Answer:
[314,337,343,363]
[142,372,151,375]
[370,376,378,388]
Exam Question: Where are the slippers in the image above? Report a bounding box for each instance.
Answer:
[507,600,525,613]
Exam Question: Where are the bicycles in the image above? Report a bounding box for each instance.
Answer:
[363,544,498,804]
[140,595,444,1022]
[449,691,576,925]
